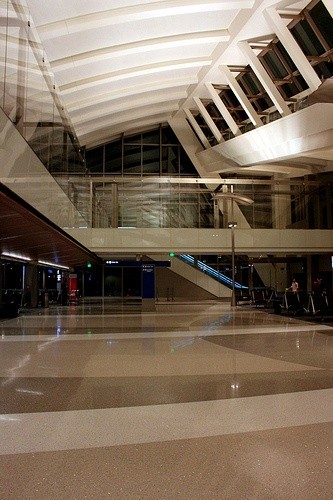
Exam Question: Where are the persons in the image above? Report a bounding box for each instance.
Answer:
[287,278,299,294]
[312,273,324,296]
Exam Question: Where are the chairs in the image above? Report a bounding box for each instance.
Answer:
[234,287,333,320]
[0,289,64,317]
